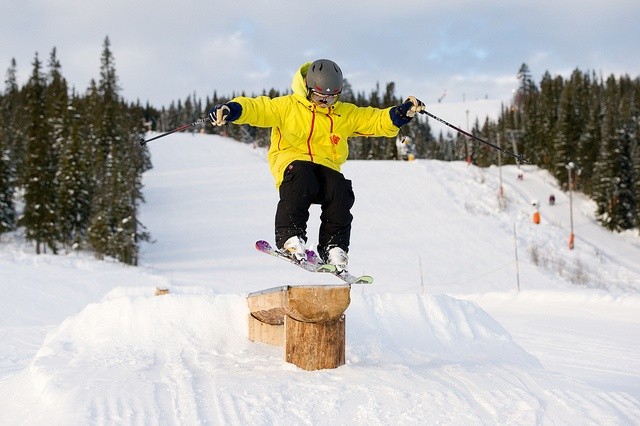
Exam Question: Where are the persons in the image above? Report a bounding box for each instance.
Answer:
[208,58,426,277]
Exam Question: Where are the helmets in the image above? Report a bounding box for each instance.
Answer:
[307,58,343,94]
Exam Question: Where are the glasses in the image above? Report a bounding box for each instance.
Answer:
[311,90,341,106]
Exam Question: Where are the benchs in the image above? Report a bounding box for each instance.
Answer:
[249,285,351,371]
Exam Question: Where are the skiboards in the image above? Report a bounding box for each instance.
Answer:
[256,240,373,284]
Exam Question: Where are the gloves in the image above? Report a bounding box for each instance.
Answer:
[395,95,426,122]
[207,103,234,127]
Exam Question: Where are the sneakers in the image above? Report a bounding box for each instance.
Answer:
[282,235,308,262]
[322,245,349,274]
